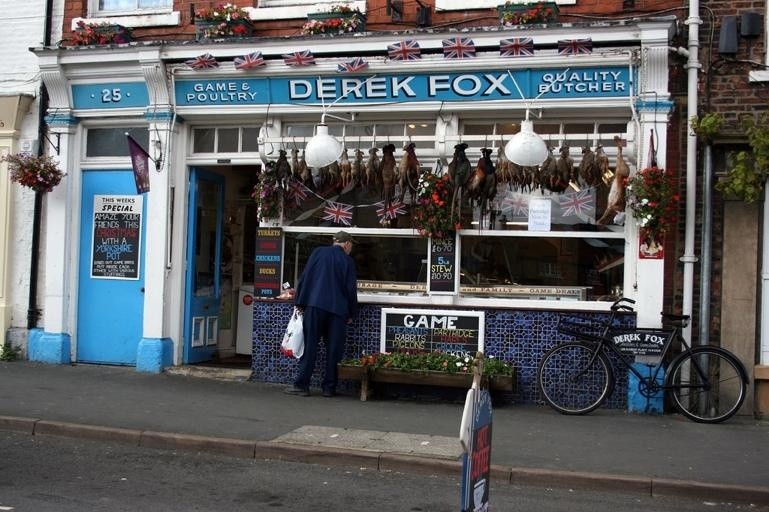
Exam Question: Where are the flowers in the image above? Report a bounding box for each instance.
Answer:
[0,151,70,194]
[499,3,552,28]
[69,18,125,47]
[250,163,281,222]
[413,173,462,241]
[622,164,683,254]
[194,2,358,40]
[340,347,515,379]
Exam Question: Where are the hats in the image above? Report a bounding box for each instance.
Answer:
[332,231,360,245]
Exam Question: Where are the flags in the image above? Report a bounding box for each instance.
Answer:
[557,37,593,56]
[559,189,594,217]
[337,56,369,73]
[184,53,219,72]
[127,134,150,194]
[441,37,477,60]
[500,190,530,218]
[499,37,535,57]
[281,49,316,67]
[283,177,312,209]
[320,200,355,226]
[375,197,409,225]
[387,39,422,62]
[234,50,266,71]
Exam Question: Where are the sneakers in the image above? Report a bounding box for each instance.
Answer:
[284,387,311,397]
[321,389,333,397]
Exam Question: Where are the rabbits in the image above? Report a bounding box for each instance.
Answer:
[596,136,630,227]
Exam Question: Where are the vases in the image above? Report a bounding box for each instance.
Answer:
[195,19,255,44]
[75,25,130,43]
[505,2,560,23]
[306,10,368,32]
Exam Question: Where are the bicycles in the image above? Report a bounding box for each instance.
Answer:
[536,297,750,424]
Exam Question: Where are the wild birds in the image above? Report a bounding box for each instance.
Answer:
[495,143,609,196]
[446,142,497,236]
[274,142,421,218]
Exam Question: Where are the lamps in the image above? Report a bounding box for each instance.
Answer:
[502,108,550,167]
[302,112,355,170]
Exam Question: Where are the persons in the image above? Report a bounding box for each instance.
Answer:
[282,232,359,397]
